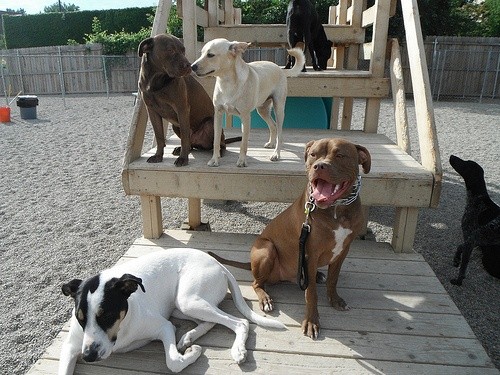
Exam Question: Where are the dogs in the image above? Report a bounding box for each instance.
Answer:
[138,33,242,167]
[60,247,288,373]
[191,38,306,168]
[284,0,334,73]
[449,155,500,286]
[250,137,372,339]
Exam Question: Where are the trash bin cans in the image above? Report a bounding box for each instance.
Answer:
[17,95,38,119]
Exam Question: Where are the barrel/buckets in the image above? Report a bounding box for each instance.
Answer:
[0,107,11,123]
